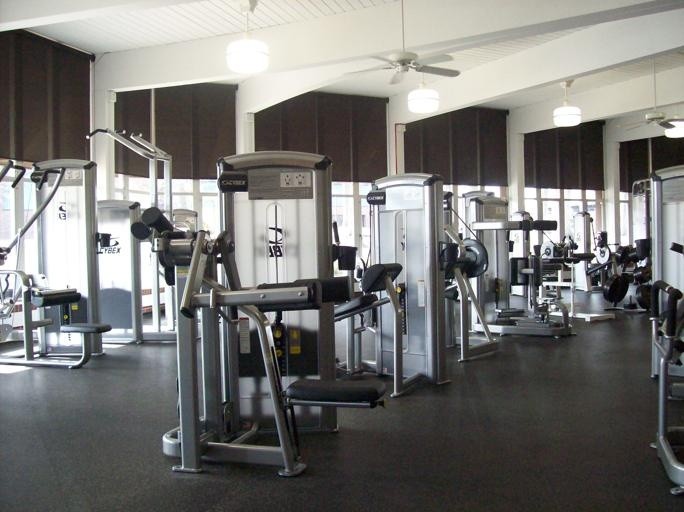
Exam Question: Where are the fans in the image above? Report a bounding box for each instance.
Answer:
[344,1,460,86]
[616,57,684,132]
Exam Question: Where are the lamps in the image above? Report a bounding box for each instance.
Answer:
[553,80,582,128]
